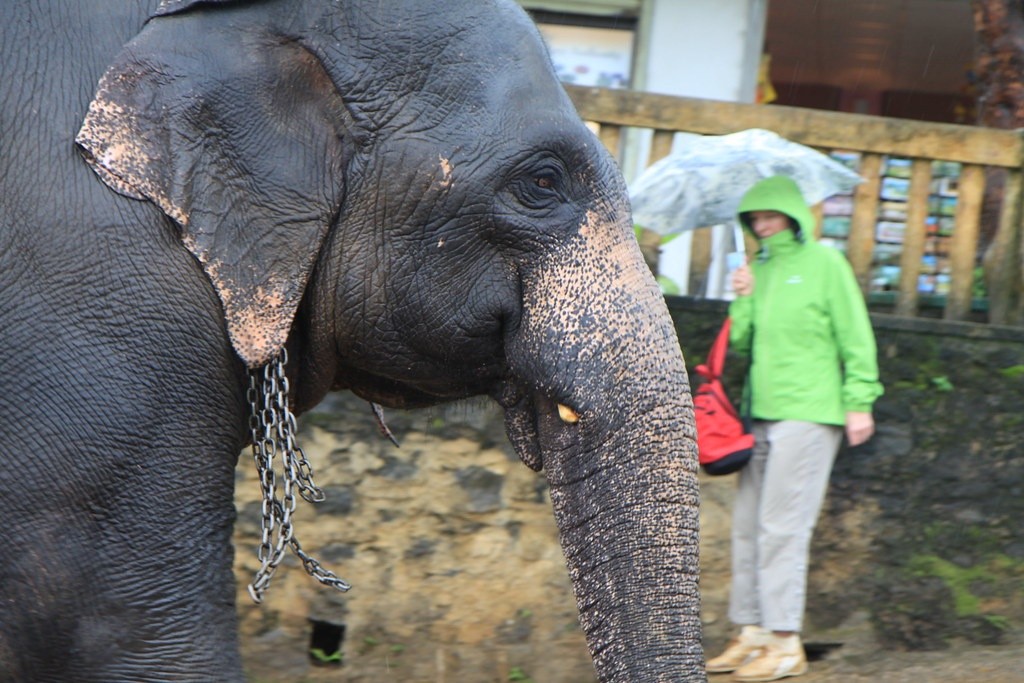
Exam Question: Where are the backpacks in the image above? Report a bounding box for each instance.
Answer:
[694,316,754,477]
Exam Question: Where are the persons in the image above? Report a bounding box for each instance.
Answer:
[705,179,886,682]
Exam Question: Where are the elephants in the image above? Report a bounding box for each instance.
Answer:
[0,0,708,683]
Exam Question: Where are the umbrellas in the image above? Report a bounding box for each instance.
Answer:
[630,128,862,231]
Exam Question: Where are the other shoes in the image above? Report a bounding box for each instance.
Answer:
[736,634,807,682]
[705,629,768,673]
